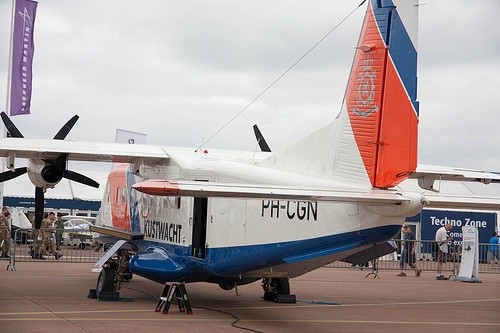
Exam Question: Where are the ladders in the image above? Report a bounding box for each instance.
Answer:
[155,281,193,315]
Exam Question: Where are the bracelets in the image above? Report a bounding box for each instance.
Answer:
[412,247,416,250]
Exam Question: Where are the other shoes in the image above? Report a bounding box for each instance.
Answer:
[416,270,421,277]
[437,274,447,280]
[396,272,406,276]
[29,245,46,260]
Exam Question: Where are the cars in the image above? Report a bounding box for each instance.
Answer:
[63,218,94,250]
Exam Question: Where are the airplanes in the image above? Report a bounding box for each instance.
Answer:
[0,0,500,316]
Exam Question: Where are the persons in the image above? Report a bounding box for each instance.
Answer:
[396,226,422,277]
[28,212,63,260]
[388,238,398,261]
[54,214,64,250]
[488,231,500,269]
[0,206,11,259]
[435,224,452,279]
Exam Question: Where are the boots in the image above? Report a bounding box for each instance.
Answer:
[2,250,10,257]
[55,253,63,260]
[46,246,52,253]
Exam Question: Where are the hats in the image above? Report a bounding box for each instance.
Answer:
[403,224,409,228]
